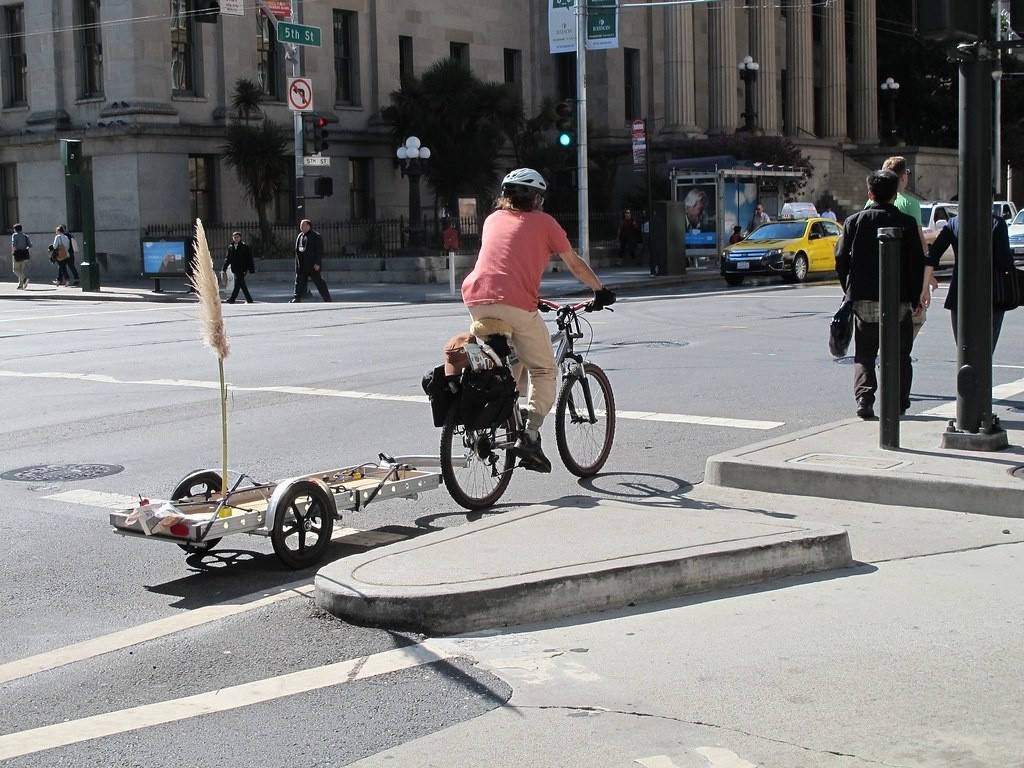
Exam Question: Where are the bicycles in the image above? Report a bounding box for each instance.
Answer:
[439,297,616,510]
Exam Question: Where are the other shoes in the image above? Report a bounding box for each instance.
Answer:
[53,281,60,285]
[65,281,70,287]
[22,278,30,290]
[221,298,235,303]
[73,281,79,286]
[17,286,23,290]
[291,298,300,303]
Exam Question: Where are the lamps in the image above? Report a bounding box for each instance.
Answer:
[788,166,793,172]
[766,164,776,171]
[778,164,786,172]
[799,167,808,172]
[753,162,763,171]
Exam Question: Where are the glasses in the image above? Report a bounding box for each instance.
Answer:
[902,169,912,175]
[756,208,761,210]
[626,212,631,214]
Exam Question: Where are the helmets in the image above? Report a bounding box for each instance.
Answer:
[500,168,547,199]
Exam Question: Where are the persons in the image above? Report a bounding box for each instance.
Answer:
[863,156,938,342]
[222,232,255,303]
[461,167,616,473]
[729,226,745,244]
[160,254,176,272]
[834,170,925,417]
[753,202,770,231]
[53,227,71,287]
[617,208,650,267]
[11,224,30,290]
[683,188,708,233]
[288,219,332,303]
[821,204,837,222]
[60,224,80,286]
[920,187,1020,356]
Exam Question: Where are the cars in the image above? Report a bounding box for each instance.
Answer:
[993,200,1017,227]
[719,202,843,287]
[1008,208,1024,272]
[917,200,961,279]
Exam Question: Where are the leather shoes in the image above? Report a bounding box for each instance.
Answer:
[856,396,874,418]
[901,406,905,415]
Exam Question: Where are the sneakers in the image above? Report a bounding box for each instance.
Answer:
[514,430,552,473]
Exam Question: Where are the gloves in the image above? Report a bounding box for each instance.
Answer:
[538,303,550,312]
[594,287,616,306]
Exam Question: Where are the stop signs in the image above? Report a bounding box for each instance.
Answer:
[632,120,645,134]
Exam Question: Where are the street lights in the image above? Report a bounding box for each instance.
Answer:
[880,78,900,146]
[396,137,430,247]
[737,55,760,126]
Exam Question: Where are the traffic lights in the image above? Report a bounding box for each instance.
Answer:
[314,177,333,196]
[555,99,579,148]
[312,117,330,152]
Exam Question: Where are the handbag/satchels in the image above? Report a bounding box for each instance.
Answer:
[57,236,67,260]
[13,249,30,262]
[422,364,460,427]
[462,366,519,430]
[829,296,853,358]
[994,269,1024,310]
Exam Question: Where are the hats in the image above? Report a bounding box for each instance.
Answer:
[444,332,478,375]
[685,189,705,207]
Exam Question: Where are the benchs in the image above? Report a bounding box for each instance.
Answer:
[686,249,718,270]
